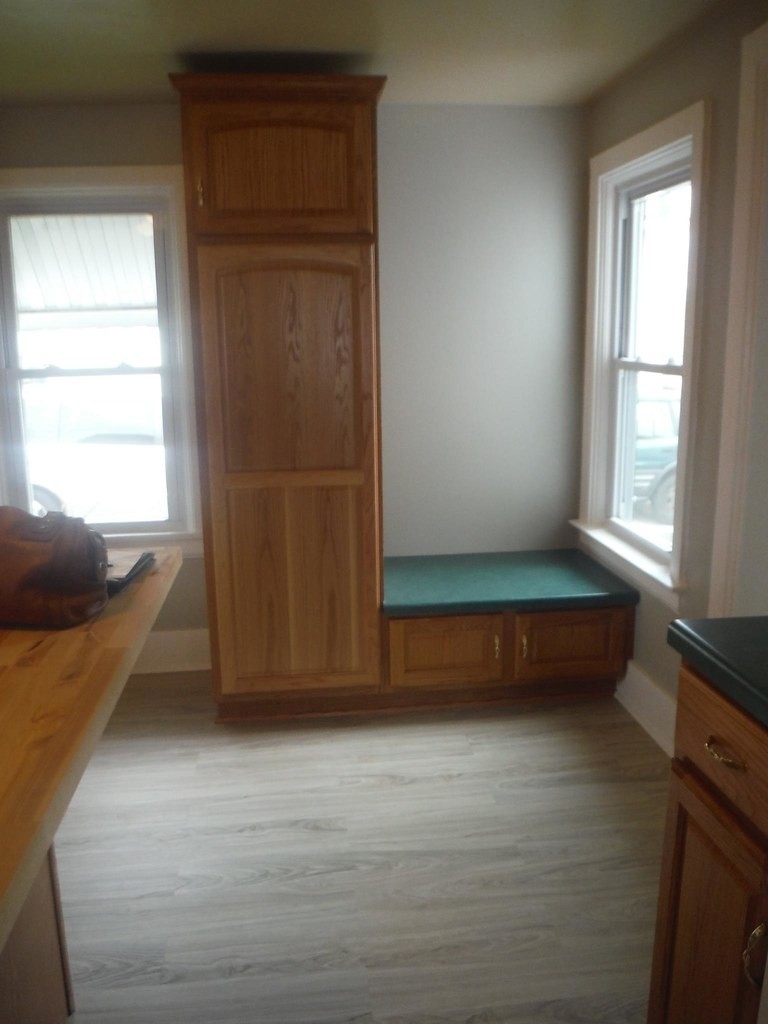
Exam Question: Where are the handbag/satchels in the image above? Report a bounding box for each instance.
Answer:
[0,504,157,630]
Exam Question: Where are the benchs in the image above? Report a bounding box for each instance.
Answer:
[384,548,640,621]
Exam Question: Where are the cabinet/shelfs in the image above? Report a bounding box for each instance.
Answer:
[637,671,767,1024]
[167,70,388,711]
[383,606,642,699]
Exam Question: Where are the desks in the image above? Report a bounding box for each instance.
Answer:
[0,545,184,1022]
